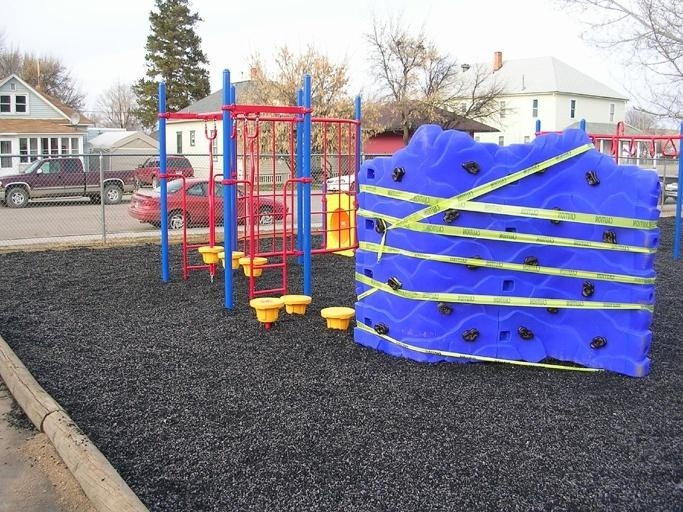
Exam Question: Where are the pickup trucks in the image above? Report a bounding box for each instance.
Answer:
[0,156,140,209]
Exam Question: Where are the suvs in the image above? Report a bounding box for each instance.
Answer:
[134,155,195,190]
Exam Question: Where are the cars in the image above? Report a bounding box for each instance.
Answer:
[323,169,359,197]
[661,181,678,202]
[126,175,290,232]
